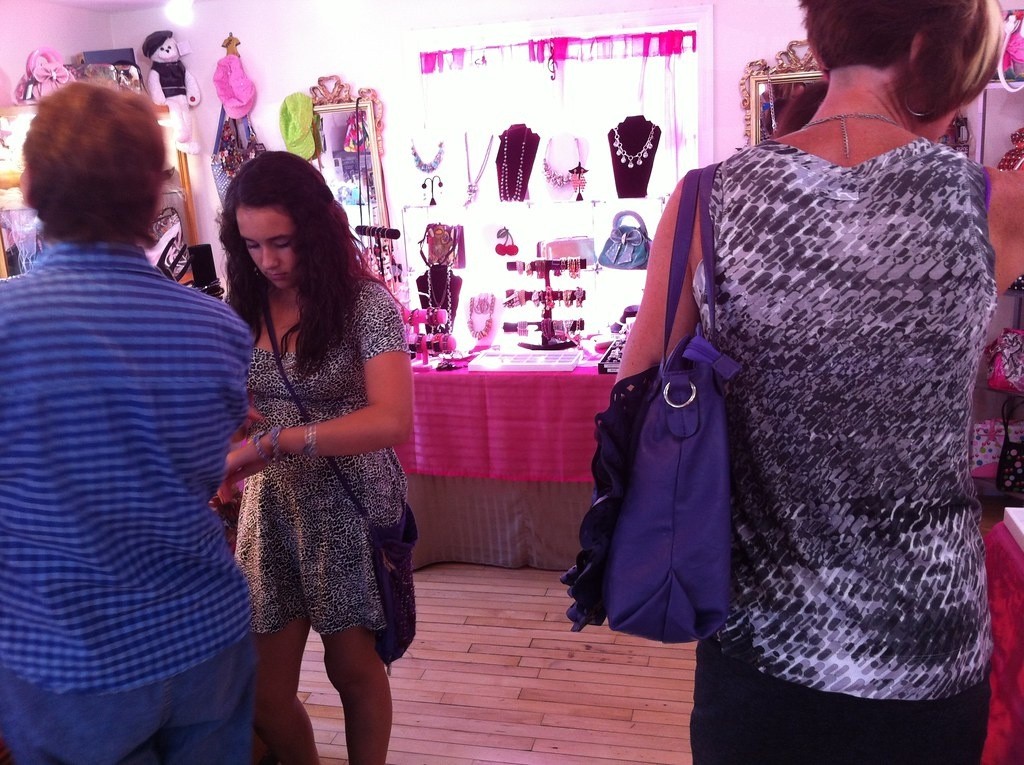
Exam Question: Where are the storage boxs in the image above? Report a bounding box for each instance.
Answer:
[468,349,583,372]
[70,48,135,64]
[597,338,626,375]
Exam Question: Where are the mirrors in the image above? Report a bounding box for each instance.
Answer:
[307,74,395,285]
[740,39,830,149]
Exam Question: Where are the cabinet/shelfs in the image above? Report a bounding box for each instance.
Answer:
[0,103,199,289]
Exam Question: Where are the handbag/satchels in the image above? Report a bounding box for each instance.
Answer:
[211,104,266,209]
[15,48,78,107]
[560,164,742,645]
[598,210,651,270]
[970,9,1024,495]
[369,505,418,676]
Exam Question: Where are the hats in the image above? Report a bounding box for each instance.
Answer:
[279,92,315,161]
[212,54,257,119]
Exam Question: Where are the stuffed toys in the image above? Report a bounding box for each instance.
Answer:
[142,30,201,155]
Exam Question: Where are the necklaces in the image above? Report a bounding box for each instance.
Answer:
[800,112,896,158]
[543,138,583,188]
[427,266,452,335]
[500,130,528,205]
[411,142,444,173]
[463,132,494,207]
[360,226,394,284]
[468,294,495,339]
[613,123,657,168]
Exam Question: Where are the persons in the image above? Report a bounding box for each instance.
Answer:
[615,0,1024,765]
[759,82,806,139]
[217,151,414,765]
[0,83,259,765]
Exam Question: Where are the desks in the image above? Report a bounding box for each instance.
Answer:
[234,347,619,575]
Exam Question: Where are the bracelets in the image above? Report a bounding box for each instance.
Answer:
[253,436,271,465]
[514,256,583,335]
[271,427,281,463]
[304,425,318,458]
[408,307,452,355]
